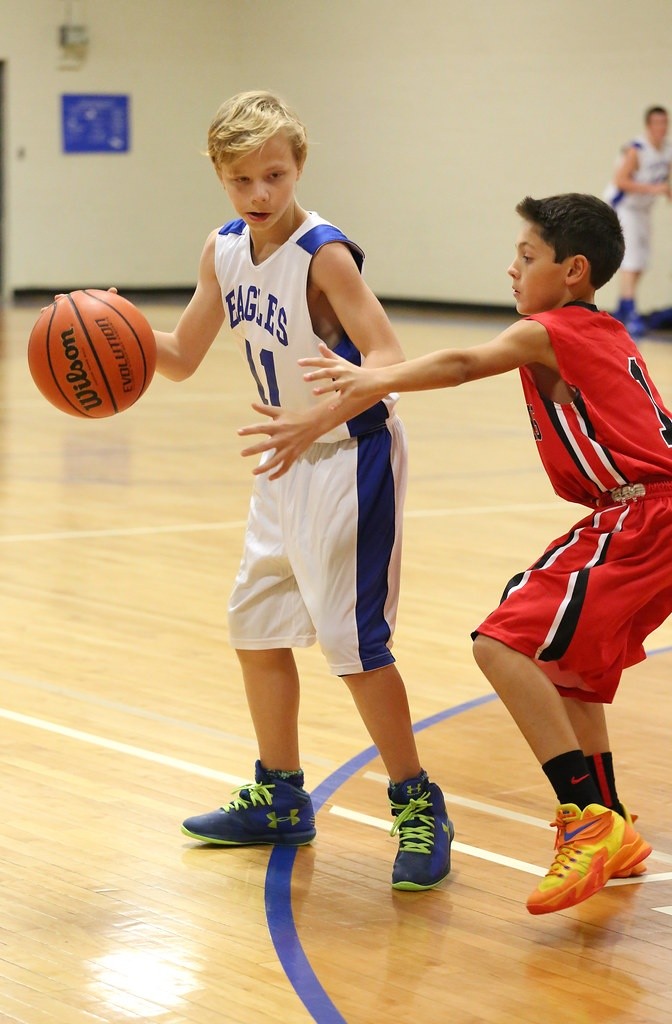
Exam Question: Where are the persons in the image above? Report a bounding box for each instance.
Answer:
[299,193,672,916]
[40,90,455,891]
[602,104,672,340]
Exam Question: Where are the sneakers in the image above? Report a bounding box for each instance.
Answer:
[615,302,648,339]
[524,798,656,914]
[180,760,319,849]
[379,769,454,889]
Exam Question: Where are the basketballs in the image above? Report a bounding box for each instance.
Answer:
[26,287,159,421]
